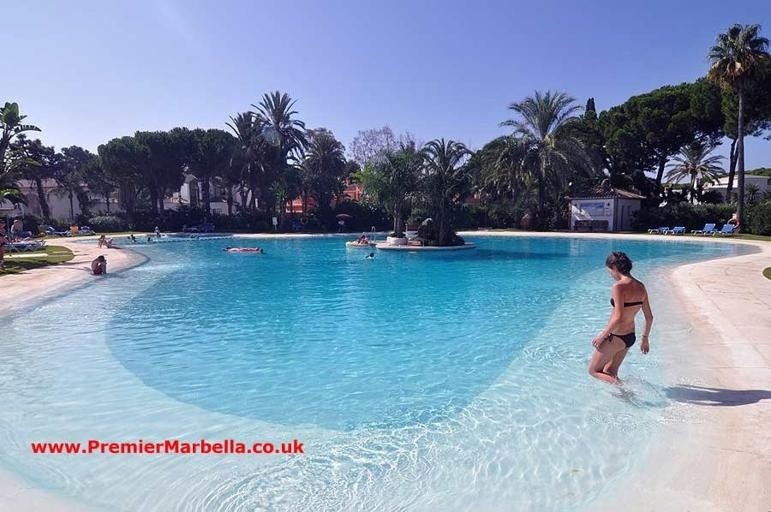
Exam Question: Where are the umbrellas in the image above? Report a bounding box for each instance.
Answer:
[335,213,353,219]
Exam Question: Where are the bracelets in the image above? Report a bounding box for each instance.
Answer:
[642,334,649,337]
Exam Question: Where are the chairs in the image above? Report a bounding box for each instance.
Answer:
[2,231,44,253]
[47,225,92,237]
[648,224,734,237]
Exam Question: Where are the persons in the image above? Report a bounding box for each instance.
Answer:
[589,250,654,389]
[337,217,346,233]
[362,253,375,259]
[128,234,137,243]
[360,235,369,244]
[147,237,152,242]
[11,217,24,243]
[96,235,125,249]
[0,219,7,268]
[91,255,107,275]
[153,225,161,238]
[222,244,264,254]
